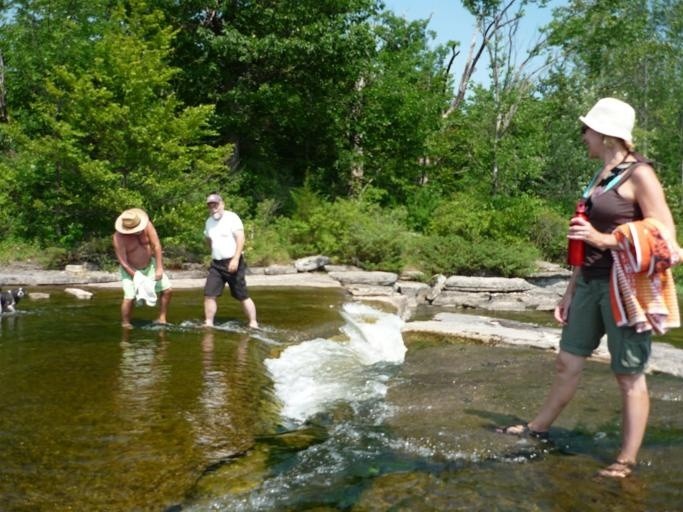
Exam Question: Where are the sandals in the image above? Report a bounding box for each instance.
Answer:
[599,461,636,478]
[495,419,549,437]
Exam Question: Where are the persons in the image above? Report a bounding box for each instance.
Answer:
[203,194,257,327]
[113,207,172,329]
[120,330,168,416]
[494,97,675,479]
[185,329,250,460]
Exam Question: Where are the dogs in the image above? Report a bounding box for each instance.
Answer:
[0,287,29,313]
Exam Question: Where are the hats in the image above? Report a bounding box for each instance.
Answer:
[206,194,223,204]
[578,97,636,139]
[114,208,149,235]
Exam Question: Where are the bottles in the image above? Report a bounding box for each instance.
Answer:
[567,199,588,268]
